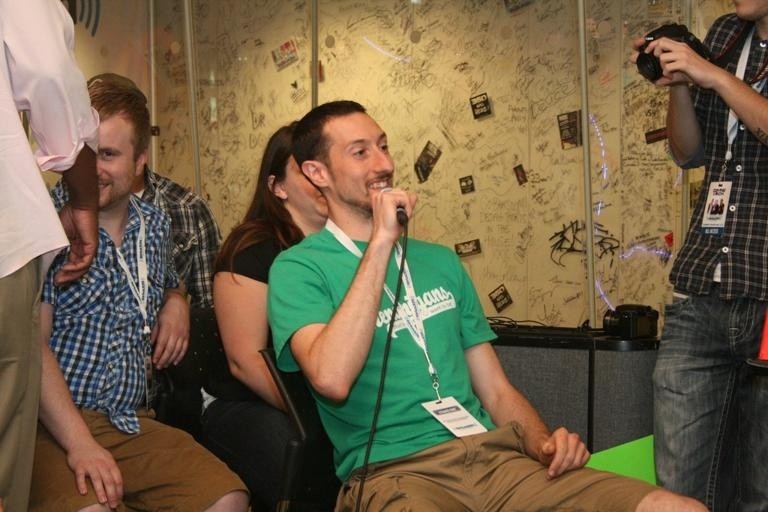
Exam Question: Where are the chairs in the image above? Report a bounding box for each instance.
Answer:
[263,348,342,510]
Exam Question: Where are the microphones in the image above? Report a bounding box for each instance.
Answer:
[379,188,409,228]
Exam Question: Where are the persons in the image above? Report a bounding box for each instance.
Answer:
[1,1,100,511]
[88,75,227,433]
[267,100,711,509]
[195,122,342,509]
[707,197,727,215]
[29,72,249,511]
[631,1,768,511]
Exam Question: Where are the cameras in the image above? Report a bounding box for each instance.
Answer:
[603,304,658,339]
[635,21,713,84]
